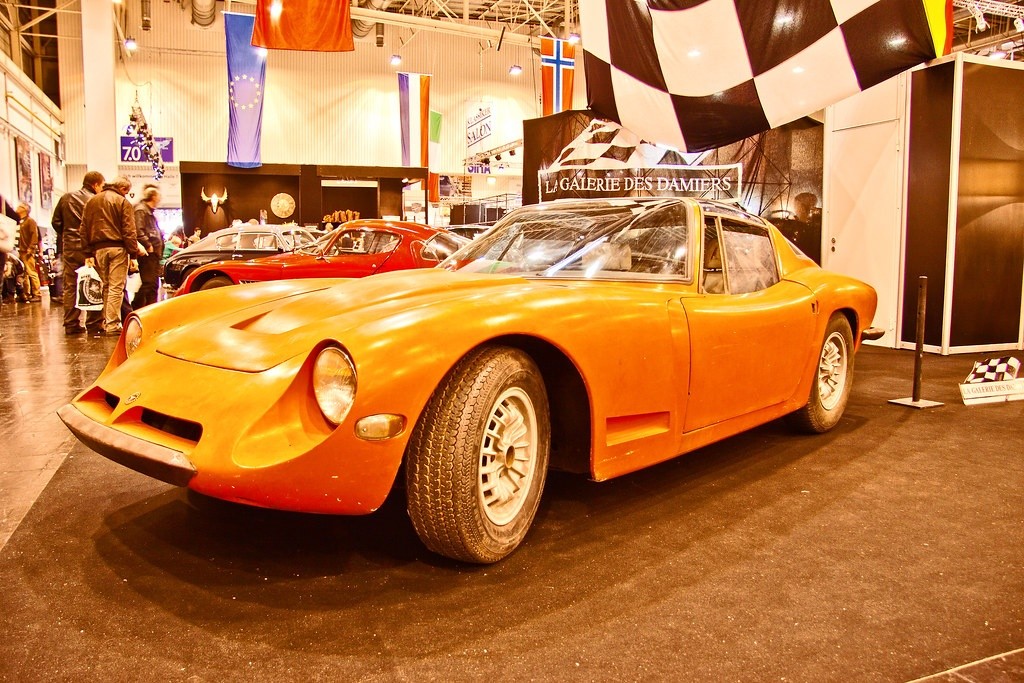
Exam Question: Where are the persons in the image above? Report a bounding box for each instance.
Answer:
[16,202,41,302]
[51,171,106,335]
[79,176,203,337]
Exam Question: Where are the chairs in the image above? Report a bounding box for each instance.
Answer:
[703,239,725,294]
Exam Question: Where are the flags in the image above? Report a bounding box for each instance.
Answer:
[540,38,575,117]
[923,0,954,58]
[429,171,440,202]
[397,73,432,168]
[250,0,354,52]
[223,13,268,168]
[429,111,443,144]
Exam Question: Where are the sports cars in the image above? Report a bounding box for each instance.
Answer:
[170,217,476,300]
[53,197,887,569]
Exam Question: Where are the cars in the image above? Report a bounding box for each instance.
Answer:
[380,222,495,254]
[162,223,331,291]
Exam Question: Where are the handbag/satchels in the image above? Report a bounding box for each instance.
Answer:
[75,263,104,310]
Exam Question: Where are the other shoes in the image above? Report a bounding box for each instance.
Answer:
[18,294,28,303]
[88,327,106,335]
[66,326,85,335]
[15,294,30,302]
[107,327,123,335]
[5,297,15,304]
[29,296,42,302]
